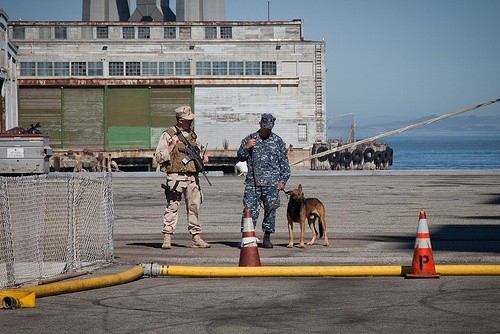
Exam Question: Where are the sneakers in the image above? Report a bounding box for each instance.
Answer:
[191,235,211,248]
[162,234,171,249]
[263,231,273,248]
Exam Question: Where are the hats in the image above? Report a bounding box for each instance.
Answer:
[174,105,195,121]
[261,113,276,130]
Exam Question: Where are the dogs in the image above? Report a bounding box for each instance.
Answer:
[285,183,330,248]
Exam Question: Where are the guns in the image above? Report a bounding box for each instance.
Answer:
[175,126,213,187]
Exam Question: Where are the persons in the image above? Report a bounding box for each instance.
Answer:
[155,106,212,249]
[237,113,290,248]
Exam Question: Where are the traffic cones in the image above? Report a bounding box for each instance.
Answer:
[238,208,262,269]
[404,210,443,278]
